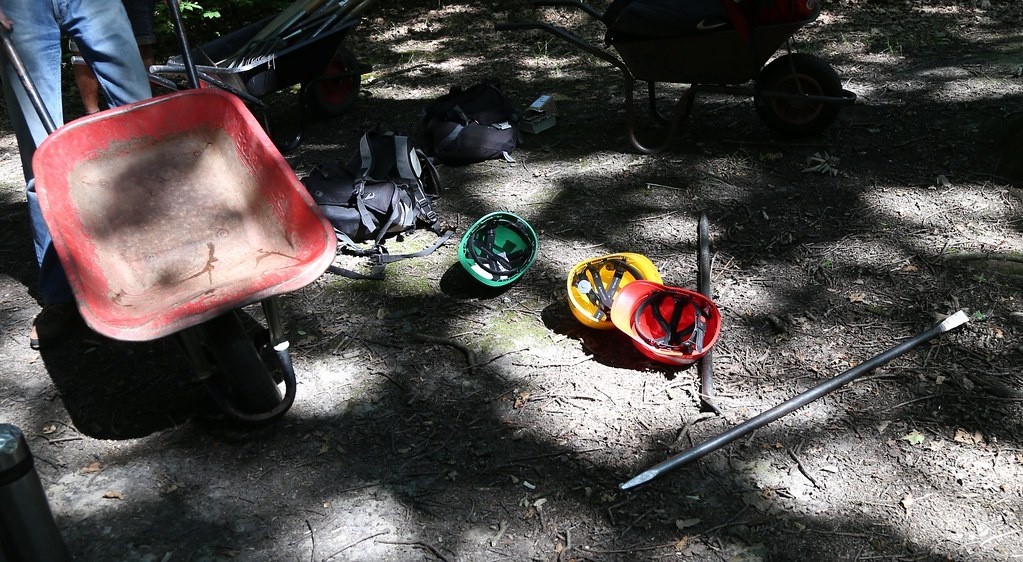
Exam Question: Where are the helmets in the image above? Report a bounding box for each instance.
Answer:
[458,210,538,287]
[611,280,722,366]
[566,253,663,330]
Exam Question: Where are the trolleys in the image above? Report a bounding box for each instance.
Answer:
[1,0,335,428]
[495,0,858,155]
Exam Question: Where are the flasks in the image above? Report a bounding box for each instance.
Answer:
[0,422,72,562]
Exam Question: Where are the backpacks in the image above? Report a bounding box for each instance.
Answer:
[298,125,457,280]
[416,81,523,167]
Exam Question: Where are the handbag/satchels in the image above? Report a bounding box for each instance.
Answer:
[601,0,733,46]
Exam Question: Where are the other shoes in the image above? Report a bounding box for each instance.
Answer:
[33,295,76,341]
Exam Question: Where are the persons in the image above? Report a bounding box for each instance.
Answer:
[0,0,155,350]
